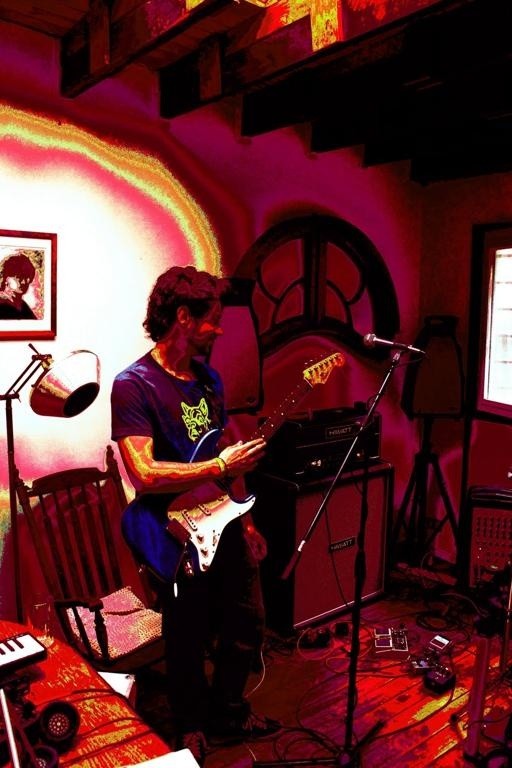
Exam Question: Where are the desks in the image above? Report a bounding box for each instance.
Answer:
[1,618,169,767]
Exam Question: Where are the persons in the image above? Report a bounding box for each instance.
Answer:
[110,266,283,748]
[0,254,40,320]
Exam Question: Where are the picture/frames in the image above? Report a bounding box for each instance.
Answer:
[0,229,58,342]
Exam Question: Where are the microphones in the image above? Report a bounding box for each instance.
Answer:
[363,333,428,356]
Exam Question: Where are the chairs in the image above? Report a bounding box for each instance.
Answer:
[11,445,169,676]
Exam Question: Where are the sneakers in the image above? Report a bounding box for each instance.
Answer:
[208,714,285,746]
[174,726,208,768]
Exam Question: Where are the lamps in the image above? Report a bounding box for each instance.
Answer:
[0,340,99,625]
[392,314,463,569]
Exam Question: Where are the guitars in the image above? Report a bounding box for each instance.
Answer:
[122,352,345,589]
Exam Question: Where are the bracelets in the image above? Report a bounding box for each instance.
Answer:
[216,457,228,477]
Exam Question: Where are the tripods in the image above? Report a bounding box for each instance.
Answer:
[281,425,386,768]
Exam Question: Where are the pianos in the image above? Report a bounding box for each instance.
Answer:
[0,631,46,674]
